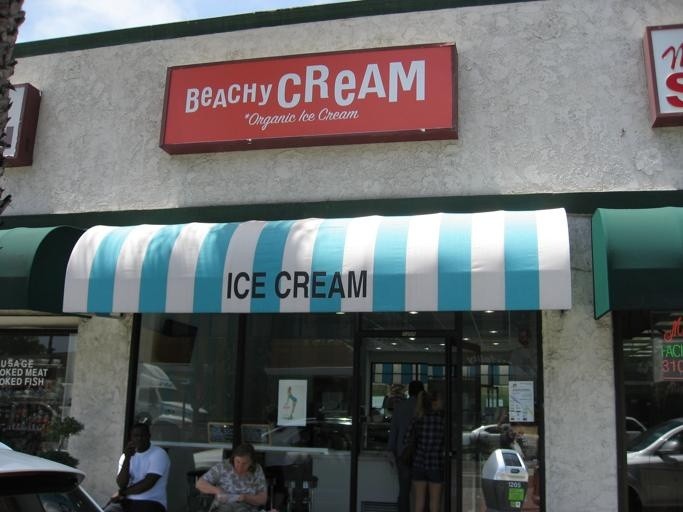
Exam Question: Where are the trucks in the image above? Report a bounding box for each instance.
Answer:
[132,361,209,441]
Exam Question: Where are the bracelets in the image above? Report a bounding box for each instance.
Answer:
[119,489,126,497]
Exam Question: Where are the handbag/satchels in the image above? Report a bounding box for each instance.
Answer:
[400,444,416,465]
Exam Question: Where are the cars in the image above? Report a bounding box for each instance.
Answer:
[625,416,683,512]
[0,442,105,512]
[193,417,400,512]
[469,424,501,446]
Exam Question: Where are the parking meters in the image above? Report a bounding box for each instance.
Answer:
[481,448,529,512]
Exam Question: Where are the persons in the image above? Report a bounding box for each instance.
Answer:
[103,423,171,511]
[496,415,513,449]
[389,380,446,512]
[196,442,268,512]
